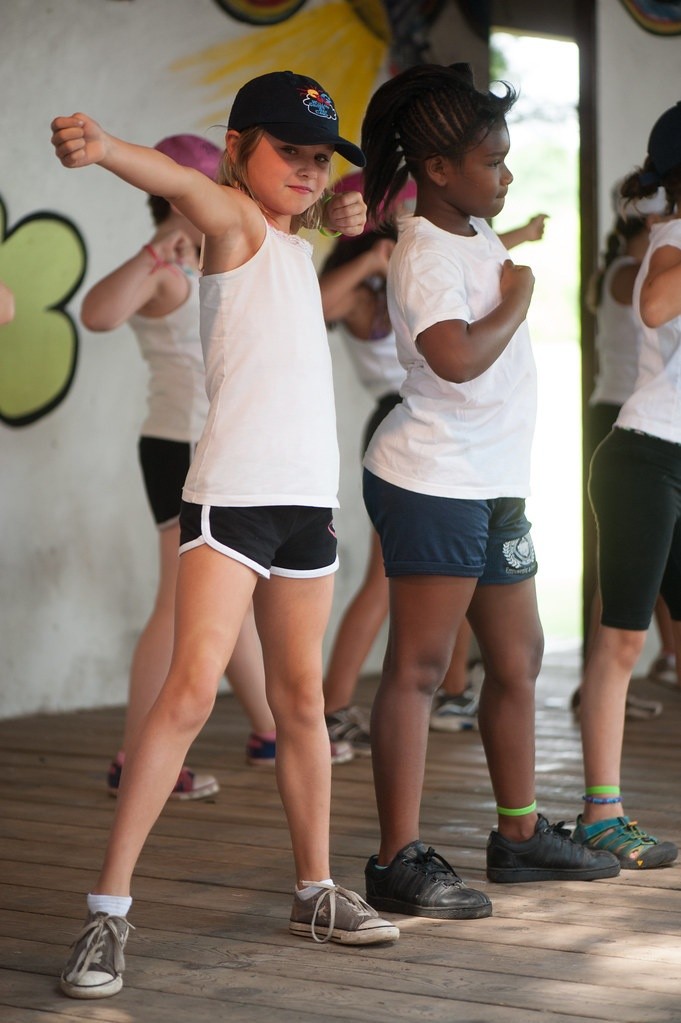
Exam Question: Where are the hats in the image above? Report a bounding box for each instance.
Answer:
[155,135,225,185]
[228,70,367,167]
[324,171,417,240]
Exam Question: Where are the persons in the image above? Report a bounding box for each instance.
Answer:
[0,133,681,802]
[59,72,398,997]
[342,60,621,920]
[566,98,681,870]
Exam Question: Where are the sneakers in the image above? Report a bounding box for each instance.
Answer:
[570,686,662,719]
[325,705,373,758]
[364,840,492,919]
[429,684,479,732]
[573,814,677,869]
[648,655,680,689]
[486,813,620,882]
[288,880,400,945]
[106,751,219,801]
[60,910,136,999]
[244,731,354,767]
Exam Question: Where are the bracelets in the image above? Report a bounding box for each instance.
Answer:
[313,196,339,237]
[141,241,172,273]
